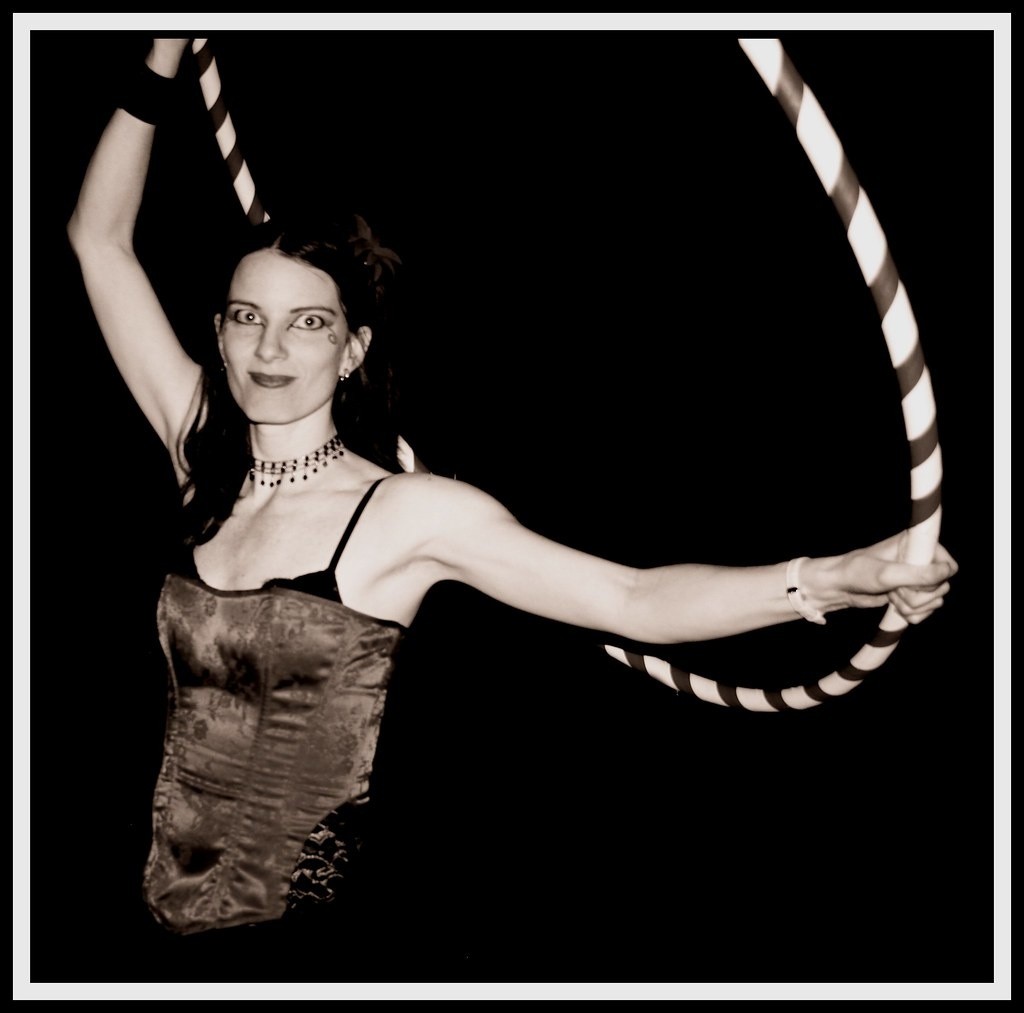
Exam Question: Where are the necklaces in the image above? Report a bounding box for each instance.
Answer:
[247,431,343,488]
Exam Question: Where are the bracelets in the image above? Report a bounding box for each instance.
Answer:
[784,556,828,627]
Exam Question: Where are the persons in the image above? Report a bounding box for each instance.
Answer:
[64,35,959,984]
